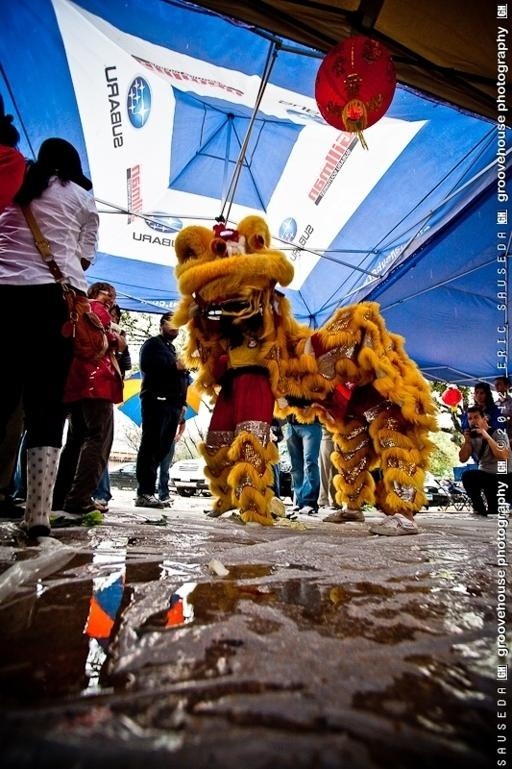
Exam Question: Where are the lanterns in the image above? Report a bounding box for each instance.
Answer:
[441,387,462,413]
[314,32,395,150]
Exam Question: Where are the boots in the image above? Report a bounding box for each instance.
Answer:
[19,446,61,537]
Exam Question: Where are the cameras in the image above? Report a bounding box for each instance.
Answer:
[468,425,483,439]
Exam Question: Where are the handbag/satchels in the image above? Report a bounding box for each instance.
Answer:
[61,295,109,362]
[114,345,131,370]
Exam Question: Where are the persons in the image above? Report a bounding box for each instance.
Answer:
[0,89,192,540]
[458,405,512,517]
[284,413,324,516]
[456,382,509,464]
[316,427,342,511]
[267,418,284,499]
[492,376,511,426]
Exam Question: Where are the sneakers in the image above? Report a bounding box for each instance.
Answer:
[0,495,25,521]
[207,505,237,517]
[324,509,365,522]
[159,495,174,507]
[246,521,262,529]
[136,494,164,509]
[94,498,107,506]
[368,513,418,535]
[63,497,109,518]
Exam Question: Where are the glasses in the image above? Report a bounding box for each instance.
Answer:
[99,290,116,300]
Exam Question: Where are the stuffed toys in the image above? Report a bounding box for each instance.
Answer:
[165,213,439,536]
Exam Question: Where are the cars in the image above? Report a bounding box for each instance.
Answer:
[168,457,213,497]
[110,463,139,491]
